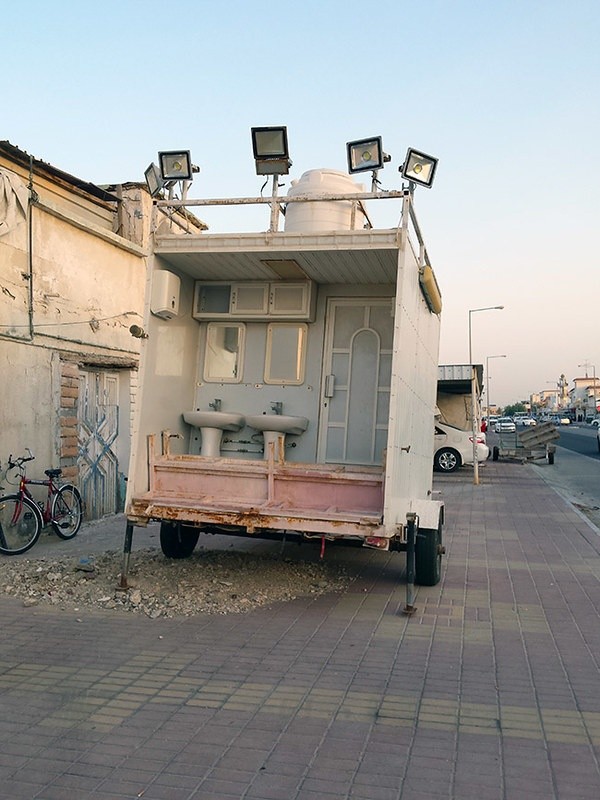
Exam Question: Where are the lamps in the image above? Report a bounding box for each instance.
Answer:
[251,126,293,168]
[144,162,177,199]
[399,148,439,188]
[158,150,200,181]
[346,135,391,175]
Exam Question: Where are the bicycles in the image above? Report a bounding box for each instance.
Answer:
[0,448,84,555]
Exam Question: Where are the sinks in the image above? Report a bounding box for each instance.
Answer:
[182,412,246,432]
[246,415,308,435]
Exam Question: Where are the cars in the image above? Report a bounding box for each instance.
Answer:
[514,416,537,427]
[494,418,517,433]
[480,415,502,426]
[433,420,490,473]
[531,412,600,428]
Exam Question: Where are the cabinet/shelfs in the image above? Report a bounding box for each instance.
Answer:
[192,280,318,324]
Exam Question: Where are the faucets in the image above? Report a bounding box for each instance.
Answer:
[270,400,282,415]
[208,399,221,411]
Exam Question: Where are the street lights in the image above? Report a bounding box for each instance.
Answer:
[486,355,507,419]
[578,364,596,412]
[468,305,504,365]
